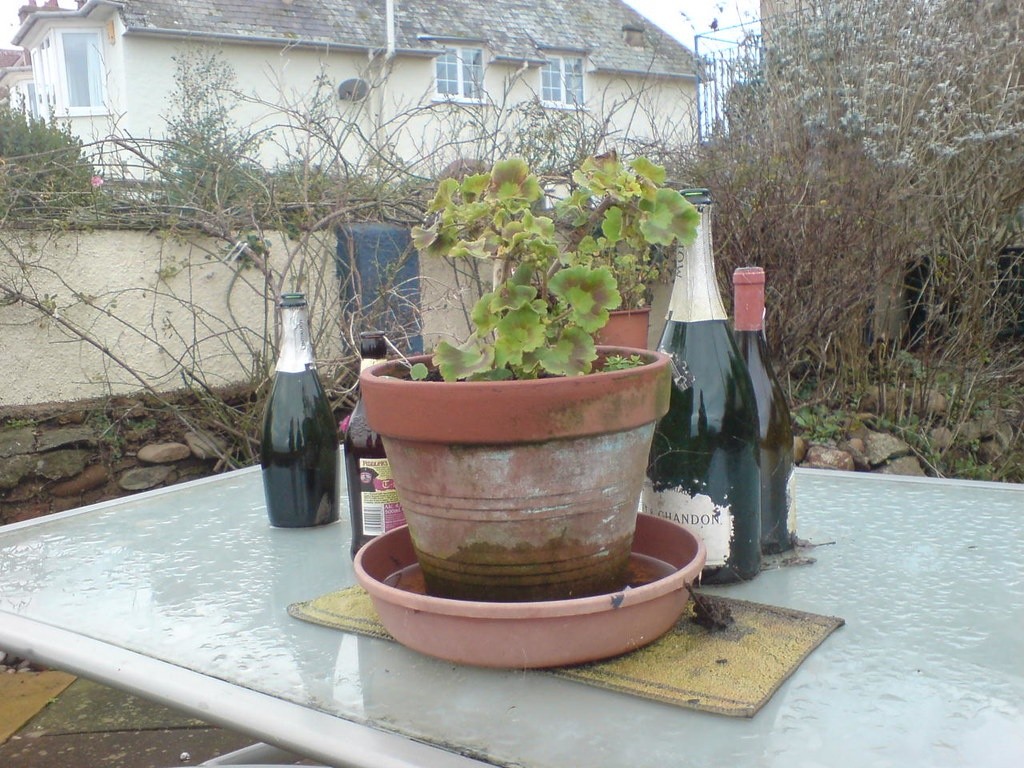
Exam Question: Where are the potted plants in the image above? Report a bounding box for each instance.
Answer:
[351,143,705,609]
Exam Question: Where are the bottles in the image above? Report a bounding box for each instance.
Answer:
[641,187,763,587]
[258,293,341,528]
[343,331,407,563]
[731,265,798,557]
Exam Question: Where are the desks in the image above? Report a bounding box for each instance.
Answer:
[0,438,1024,768]
